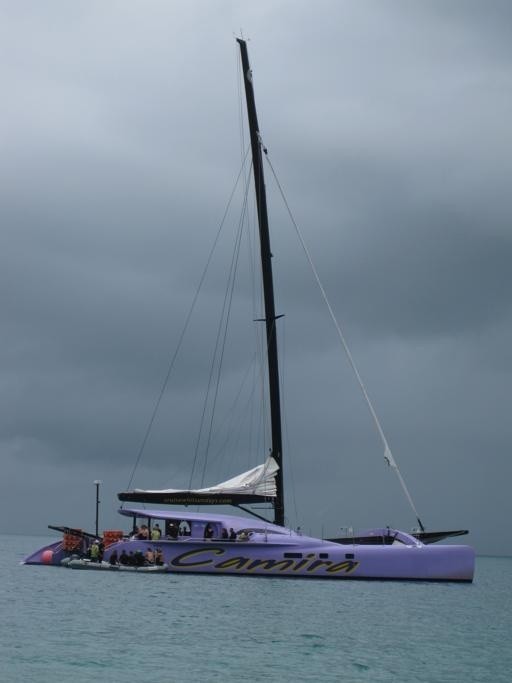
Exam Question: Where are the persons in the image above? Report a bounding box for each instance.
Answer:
[230,528,248,542]
[128,520,192,540]
[205,527,213,537]
[87,539,104,563]
[222,528,228,539]
[108,545,164,566]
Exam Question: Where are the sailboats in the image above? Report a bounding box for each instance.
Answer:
[21,36,475,585]
